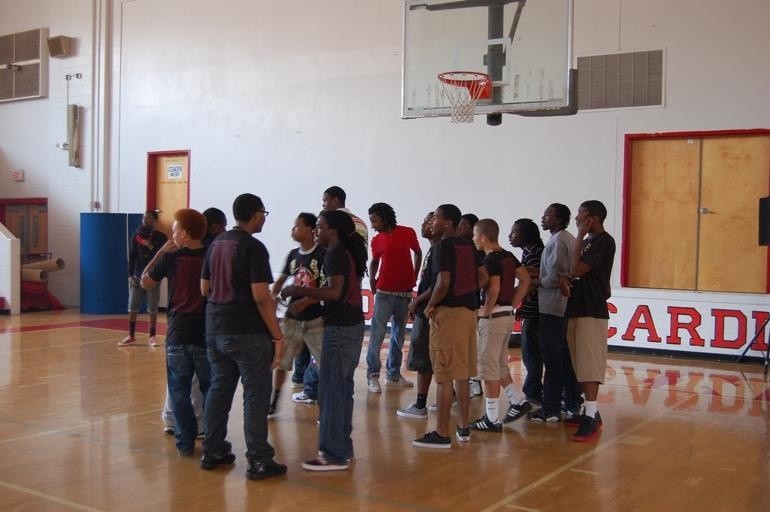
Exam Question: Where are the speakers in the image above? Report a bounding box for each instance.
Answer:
[759,198,770,246]
[47,36,70,58]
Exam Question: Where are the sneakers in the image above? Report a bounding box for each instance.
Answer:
[366,372,382,393]
[245,456,287,481]
[429,394,459,411]
[264,375,318,419]
[468,378,484,399]
[470,414,502,433]
[396,403,428,419]
[411,430,450,449]
[524,383,583,423]
[562,404,603,442]
[148,336,160,346]
[117,335,136,346]
[302,449,351,471]
[161,425,236,470]
[455,424,471,441]
[384,375,413,387]
[503,400,532,424]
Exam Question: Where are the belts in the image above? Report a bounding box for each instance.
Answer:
[478,309,515,319]
[376,289,412,298]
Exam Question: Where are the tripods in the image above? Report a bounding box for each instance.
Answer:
[737,317,770,374]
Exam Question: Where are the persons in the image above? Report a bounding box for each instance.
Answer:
[139,186,613,478]
[116,208,162,349]
